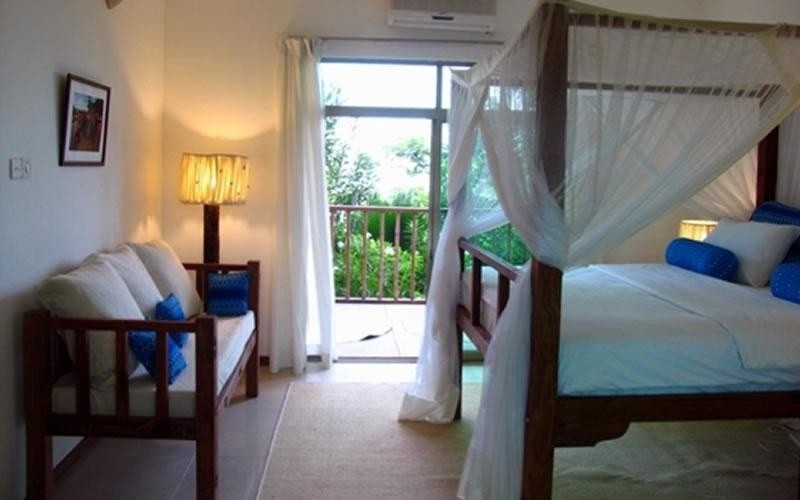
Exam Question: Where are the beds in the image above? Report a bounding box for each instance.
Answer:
[396,0,799,500]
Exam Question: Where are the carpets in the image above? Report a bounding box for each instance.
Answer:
[256,380,800,500]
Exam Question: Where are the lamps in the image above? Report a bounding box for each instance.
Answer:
[679,221,717,242]
[178,153,250,265]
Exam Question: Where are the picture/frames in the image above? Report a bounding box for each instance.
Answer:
[58,72,111,166]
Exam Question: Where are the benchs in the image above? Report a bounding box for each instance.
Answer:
[23,235,260,499]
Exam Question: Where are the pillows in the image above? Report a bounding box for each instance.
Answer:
[666,238,737,279]
[748,200,800,224]
[205,271,248,316]
[704,215,800,288]
[770,262,800,305]
[36,236,205,392]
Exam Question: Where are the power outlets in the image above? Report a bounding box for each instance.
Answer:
[11,157,23,179]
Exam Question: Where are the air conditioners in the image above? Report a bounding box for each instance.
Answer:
[386,0,497,39]
